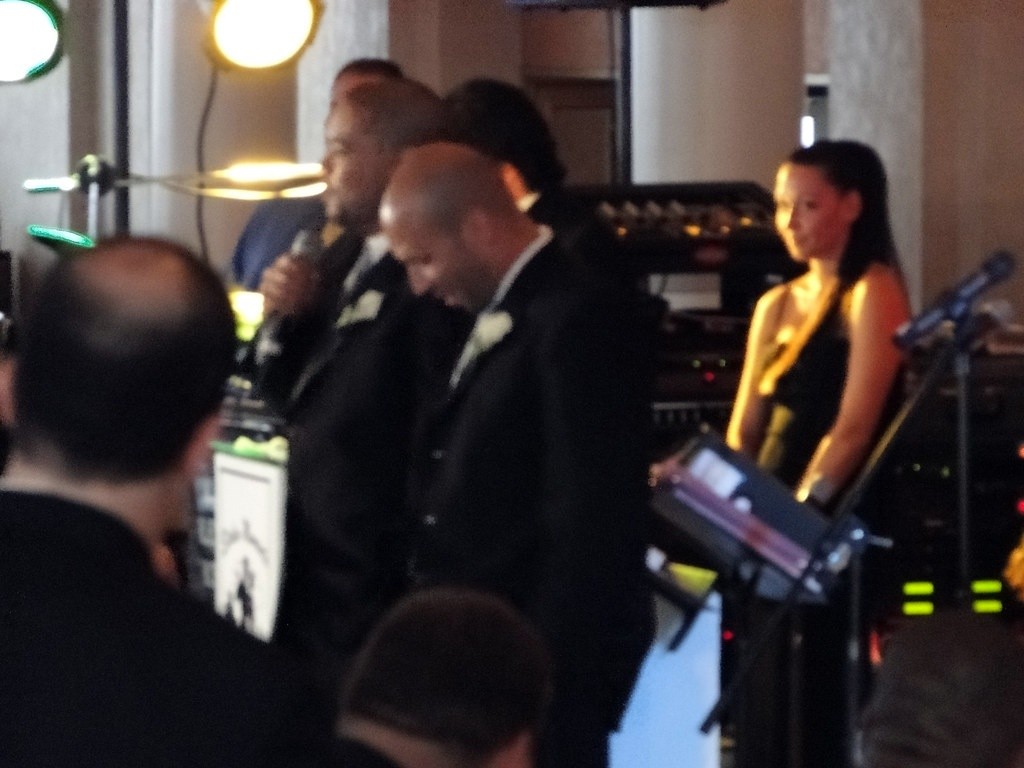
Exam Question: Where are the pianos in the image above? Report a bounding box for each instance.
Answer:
[574,178,796,444]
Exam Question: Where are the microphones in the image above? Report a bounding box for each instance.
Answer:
[891,251,1016,348]
[255,227,325,366]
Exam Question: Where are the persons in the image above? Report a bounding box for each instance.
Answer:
[0,234,345,767]
[228,57,654,768]
[721,138,913,768]
[863,611,1022,768]
